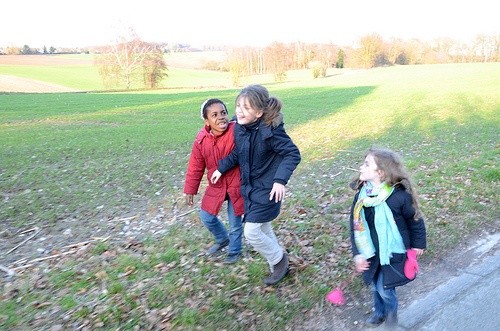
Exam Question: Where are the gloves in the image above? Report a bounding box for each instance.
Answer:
[404,249,418,280]
[326,289,344,306]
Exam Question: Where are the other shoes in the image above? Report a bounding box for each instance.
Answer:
[210,238,230,253]
[366,310,385,327]
[223,253,242,264]
[385,300,398,322]
[264,266,288,285]
[273,252,288,278]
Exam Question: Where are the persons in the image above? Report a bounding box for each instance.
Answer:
[210,84,301,283]
[349,147,426,328]
[184,98,244,264]
